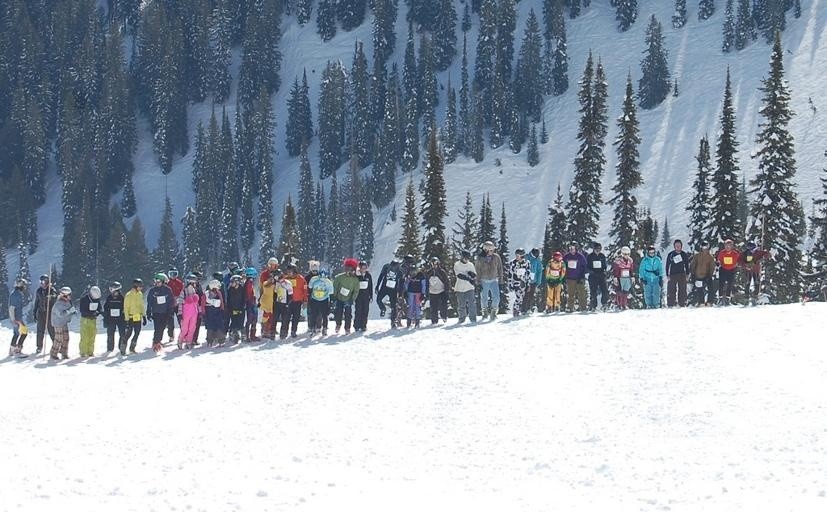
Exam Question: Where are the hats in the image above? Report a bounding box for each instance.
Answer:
[724,239,734,245]
[309,260,319,271]
[344,259,358,270]
[319,267,328,275]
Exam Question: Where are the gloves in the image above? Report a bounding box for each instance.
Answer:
[613,277,618,286]
[631,276,636,285]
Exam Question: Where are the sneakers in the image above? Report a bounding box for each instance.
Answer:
[391,311,498,329]
[380,310,385,317]
[513,299,758,316]
[9,325,367,361]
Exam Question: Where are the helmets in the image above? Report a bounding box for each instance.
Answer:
[622,246,631,257]
[209,262,258,290]
[14,279,28,292]
[359,261,368,269]
[648,245,655,250]
[389,241,602,276]
[58,287,72,300]
[108,281,122,296]
[287,263,297,273]
[38,274,50,288]
[89,286,102,299]
[268,258,279,268]
[272,269,283,282]
[154,267,204,283]
[132,278,145,292]
[700,240,710,250]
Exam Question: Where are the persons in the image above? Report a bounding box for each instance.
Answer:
[716,239,741,306]
[78,285,103,359]
[119,278,146,359]
[612,246,635,310]
[257,256,373,341]
[49,287,78,360]
[507,243,611,314]
[33,275,57,353]
[8,278,28,359]
[689,246,715,307]
[147,261,261,352]
[737,241,767,307]
[375,241,502,330]
[666,239,689,309]
[103,281,124,357]
[639,247,664,309]
